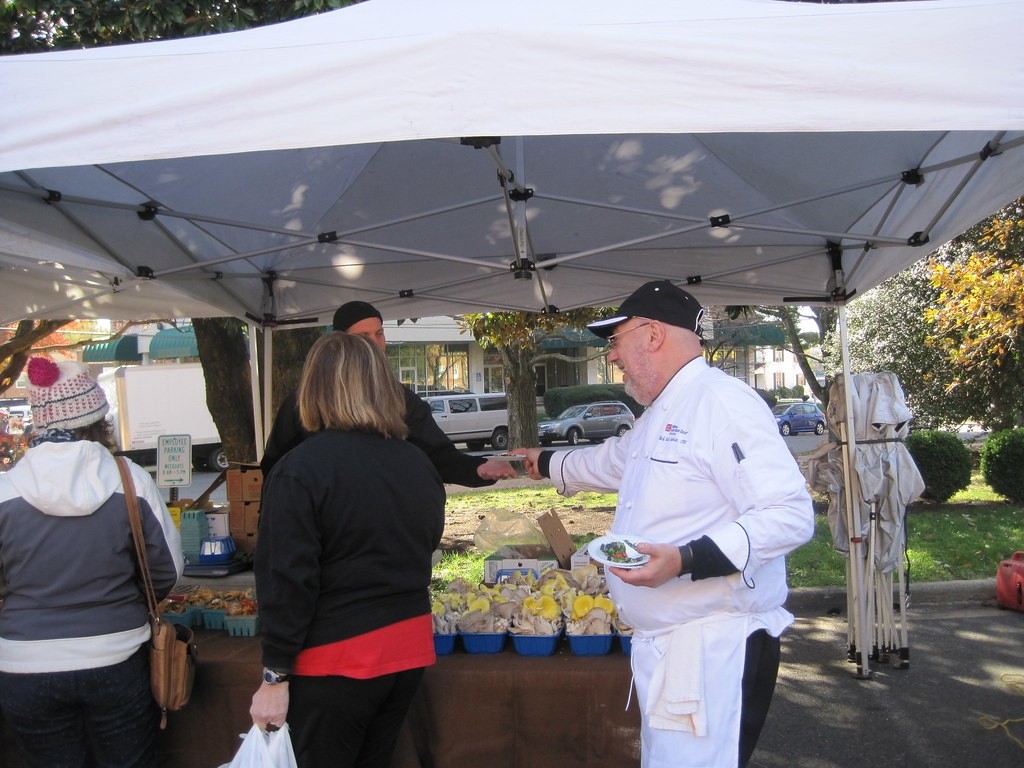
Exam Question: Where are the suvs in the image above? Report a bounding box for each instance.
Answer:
[538,399,636,447]
[417,391,509,451]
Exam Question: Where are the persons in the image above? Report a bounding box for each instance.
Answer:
[0,354,185,768]
[260,302,520,489]
[521,278,814,768]
[249,330,446,768]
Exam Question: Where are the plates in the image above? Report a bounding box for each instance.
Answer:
[588,534,656,569]
[482,452,527,462]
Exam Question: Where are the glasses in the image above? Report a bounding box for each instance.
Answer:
[606,320,652,349]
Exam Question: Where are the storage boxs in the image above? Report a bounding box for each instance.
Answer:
[161,470,638,656]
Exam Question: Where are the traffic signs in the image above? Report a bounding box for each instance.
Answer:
[156,433,193,489]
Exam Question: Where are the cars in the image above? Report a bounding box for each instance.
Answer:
[770,397,828,436]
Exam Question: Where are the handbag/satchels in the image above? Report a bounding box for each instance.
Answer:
[220,718,298,768]
[148,614,197,712]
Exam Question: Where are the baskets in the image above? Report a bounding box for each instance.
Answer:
[156,592,261,636]
[433,615,632,655]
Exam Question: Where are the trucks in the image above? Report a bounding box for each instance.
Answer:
[97,357,230,474]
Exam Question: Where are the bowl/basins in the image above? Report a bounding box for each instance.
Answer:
[427,632,638,655]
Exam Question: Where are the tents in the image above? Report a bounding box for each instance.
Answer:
[811,370,927,680]
[0,0,1023,678]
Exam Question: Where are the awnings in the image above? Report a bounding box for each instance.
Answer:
[148,325,200,365]
[538,325,610,349]
[707,322,785,346]
[82,333,143,365]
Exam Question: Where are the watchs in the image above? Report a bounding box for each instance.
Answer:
[263,668,288,685]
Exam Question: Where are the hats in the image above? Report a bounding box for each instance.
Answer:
[25,357,110,429]
[588,281,704,340]
[333,301,384,333]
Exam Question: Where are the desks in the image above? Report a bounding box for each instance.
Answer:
[153,628,642,767]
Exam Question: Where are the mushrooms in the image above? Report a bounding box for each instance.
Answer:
[427,565,635,636]
